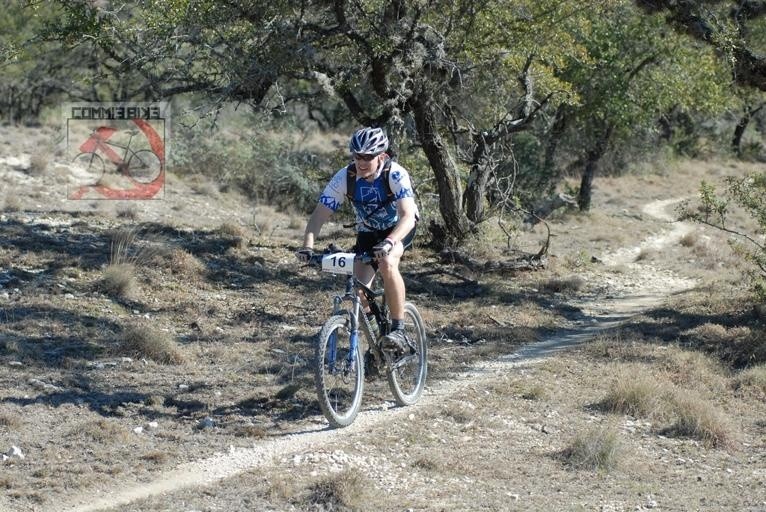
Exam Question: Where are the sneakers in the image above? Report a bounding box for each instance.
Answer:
[381,330,411,353]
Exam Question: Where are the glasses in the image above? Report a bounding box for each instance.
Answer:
[354,153,376,161]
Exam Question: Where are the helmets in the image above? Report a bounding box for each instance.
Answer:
[348,126,390,154]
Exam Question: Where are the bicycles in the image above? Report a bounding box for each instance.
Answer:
[299,244,428,427]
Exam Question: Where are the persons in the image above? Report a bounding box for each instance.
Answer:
[295,127,421,377]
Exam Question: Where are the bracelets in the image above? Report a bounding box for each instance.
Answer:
[383,236,397,247]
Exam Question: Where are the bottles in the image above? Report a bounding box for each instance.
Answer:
[366,313,381,340]
